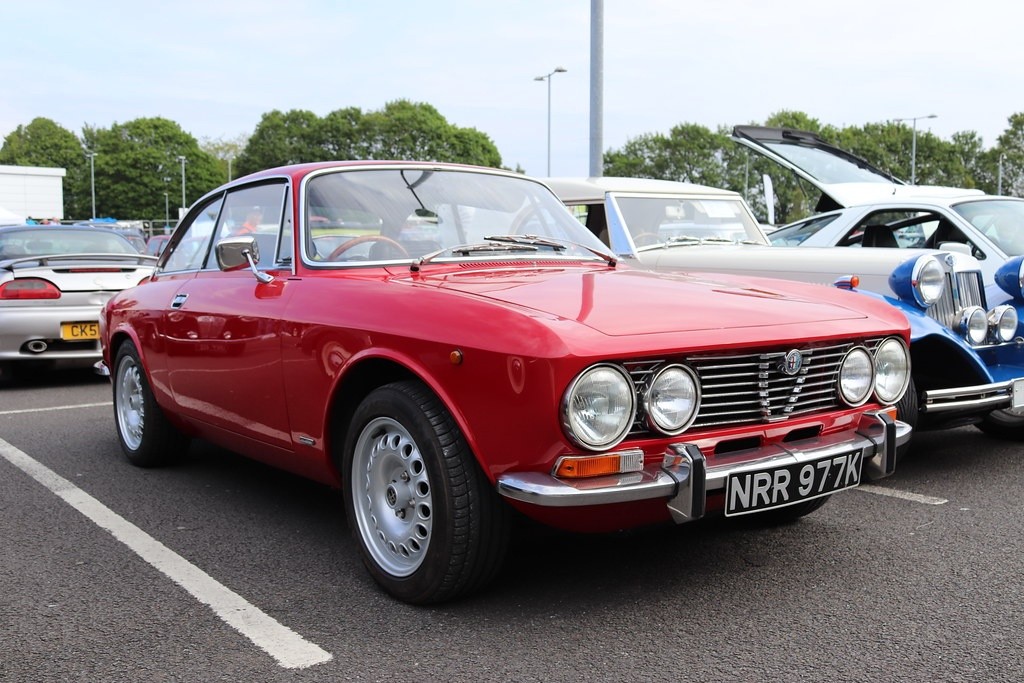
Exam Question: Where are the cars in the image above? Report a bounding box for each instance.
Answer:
[96,159,916,606]
[118,229,211,275]
[725,124,1024,254]
[539,178,1024,439]
[0,224,160,380]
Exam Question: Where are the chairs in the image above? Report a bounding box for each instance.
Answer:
[862,225,900,248]
[0,245,27,258]
[934,217,970,252]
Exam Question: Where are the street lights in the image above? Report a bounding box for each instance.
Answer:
[82,144,98,219]
[892,114,938,186]
[535,67,567,176]
